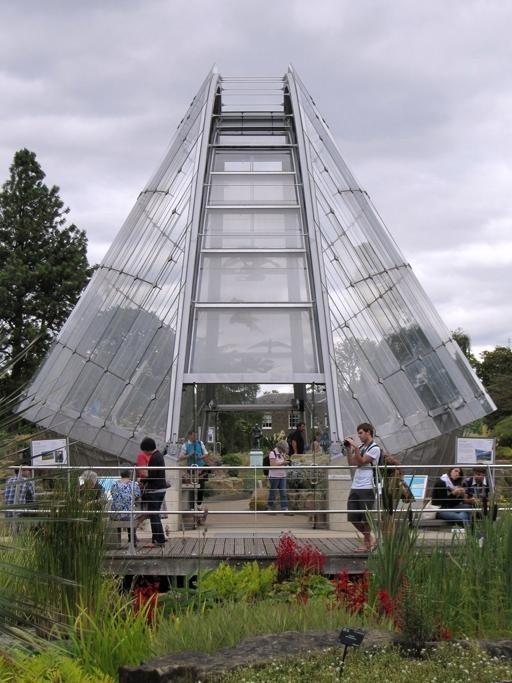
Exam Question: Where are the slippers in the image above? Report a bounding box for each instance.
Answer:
[366,537,379,553]
[354,547,367,553]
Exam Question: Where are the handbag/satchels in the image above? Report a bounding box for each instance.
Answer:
[421,501,442,520]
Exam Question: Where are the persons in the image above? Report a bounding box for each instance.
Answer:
[110,462,141,544]
[286,422,306,465]
[344,423,378,553]
[179,430,209,511]
[266,439,295,516]
[135,437,166,548]
[5,461,43,537]
[311,432,323,454]
[430,467,498,540]
[80,470,109,548]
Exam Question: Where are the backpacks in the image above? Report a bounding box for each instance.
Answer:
[263,450,276,475]
[359,444,385,484]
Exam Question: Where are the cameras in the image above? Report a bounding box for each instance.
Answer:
[286,459,292,466]
[344,437,353,447]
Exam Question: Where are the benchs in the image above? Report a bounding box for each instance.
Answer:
[98,516,137,550]
[372,499,461,540]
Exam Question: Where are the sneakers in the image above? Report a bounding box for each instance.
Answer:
[143,542,162,548]
[284,512,293,516]
